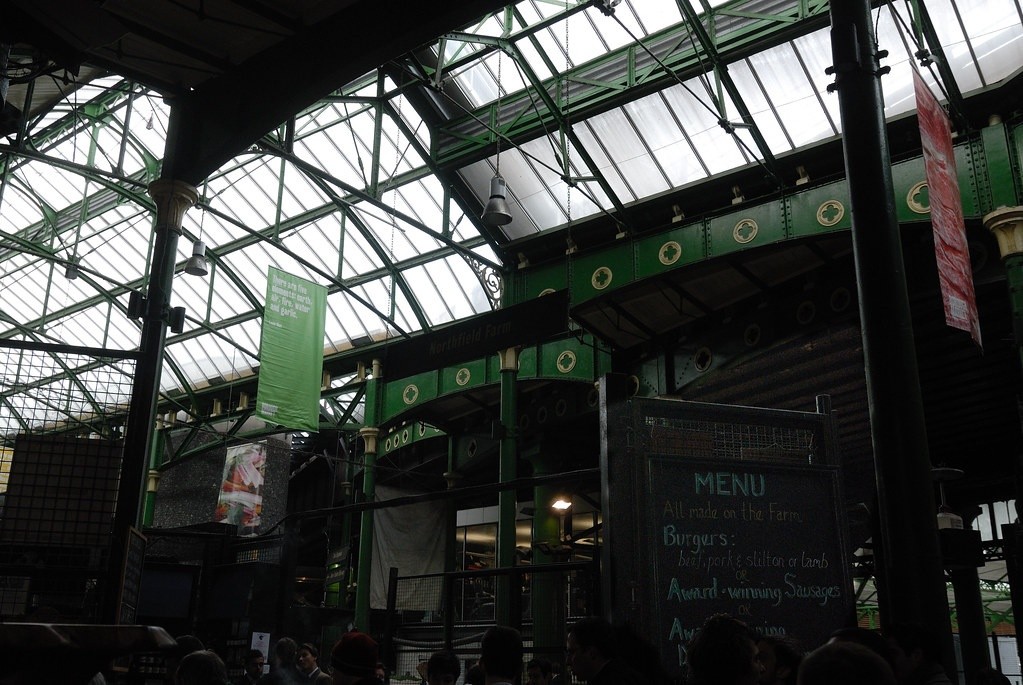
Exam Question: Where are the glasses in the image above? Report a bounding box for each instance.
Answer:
[328,664,335,674]
[887,649,920,659]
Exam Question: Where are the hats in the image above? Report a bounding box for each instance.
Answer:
[331,632,378,678]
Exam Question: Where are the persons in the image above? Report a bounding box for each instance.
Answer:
[109,614,1010,685]
